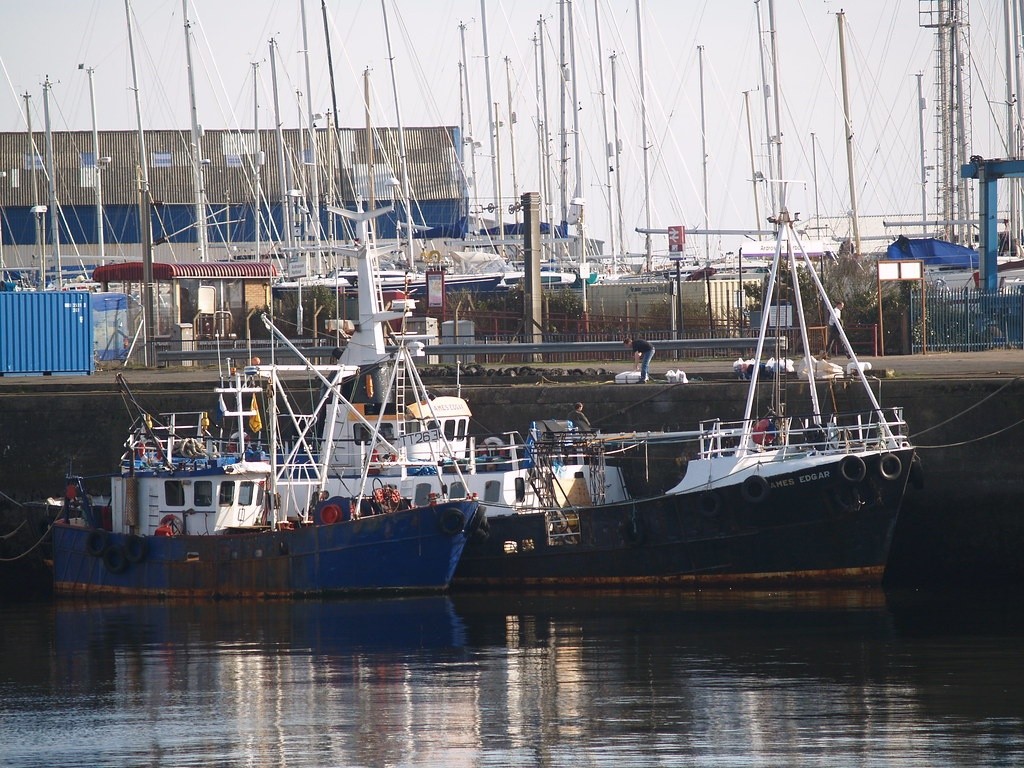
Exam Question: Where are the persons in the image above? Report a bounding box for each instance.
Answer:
[624,337,655,383]
[568,402,590,430]
[822,301,858,360]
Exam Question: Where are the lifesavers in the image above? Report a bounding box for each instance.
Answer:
[620,517,648,546]
[85,527,147,574]
[480,436,505,457]
[805,424,822,445]
[839,455,867,484]
[438,507,465,536]
[417,363,611,378]
[878,453,903,481]
[910,459,925,490]
[370,443,397,472]
[695,490,723,518]
[161,514,184,537]
[470,506,487,532]
[138,434,164,461]
[741,474,771,508]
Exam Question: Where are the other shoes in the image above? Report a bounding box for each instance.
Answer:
[636,380,646,384]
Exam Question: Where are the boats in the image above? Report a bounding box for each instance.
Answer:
[47,361,487,594]
[116,177,920,625]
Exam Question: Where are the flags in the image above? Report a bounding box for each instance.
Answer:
[249,394,262,433]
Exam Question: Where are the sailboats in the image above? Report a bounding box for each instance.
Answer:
[267,0,774,292]
